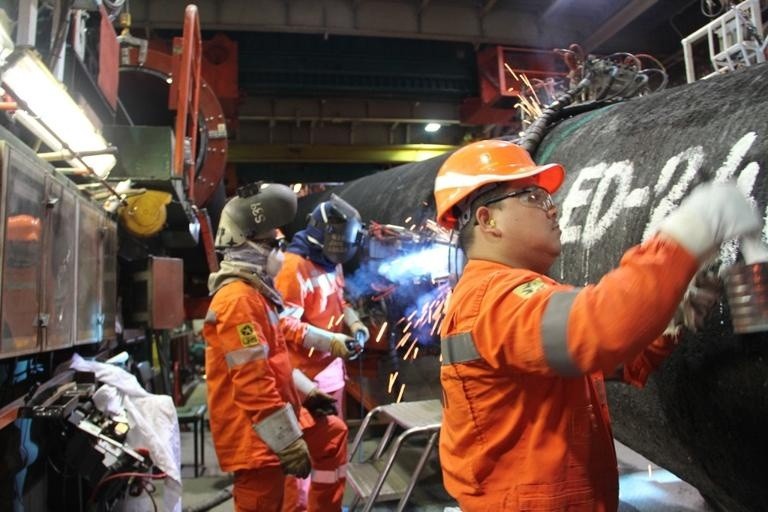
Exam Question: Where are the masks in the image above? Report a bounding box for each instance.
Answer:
[265,247,284,279]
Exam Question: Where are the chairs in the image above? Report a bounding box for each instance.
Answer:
[134,360,207,478]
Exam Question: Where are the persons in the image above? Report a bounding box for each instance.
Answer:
[435,138,765,511]
[273,196,369,510]
[204,179,352,511]
[2,215,60,353]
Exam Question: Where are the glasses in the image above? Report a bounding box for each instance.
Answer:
[253,238,286,252]
[481,185,553,212]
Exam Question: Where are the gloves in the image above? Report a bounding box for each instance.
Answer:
[302,386,337,418]
[251,435,311,479]
[658,181,760,266]
[332,324,360,361]
[343,321,369,344]
[674,269,718,330]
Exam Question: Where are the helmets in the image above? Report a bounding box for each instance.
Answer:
[214,180,297,248]
[304,193,369,263]
[434,139,564,229]
